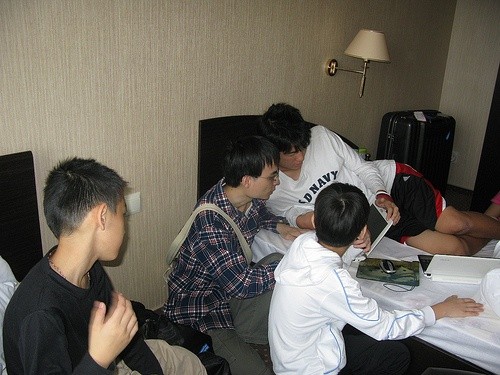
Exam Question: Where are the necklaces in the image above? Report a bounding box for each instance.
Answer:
[48,249,90,288]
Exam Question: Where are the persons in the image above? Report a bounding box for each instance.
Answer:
[260,104,500,256]
[268,183,484,375]
[485,192,500,222]
[3,156,206,375]
[166,136,311,375]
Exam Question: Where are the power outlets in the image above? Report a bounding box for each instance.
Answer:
[125,192,142,216]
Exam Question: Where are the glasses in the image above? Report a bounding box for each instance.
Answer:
[256,168,280,181]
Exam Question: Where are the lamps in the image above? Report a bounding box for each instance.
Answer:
[325,29,391,98]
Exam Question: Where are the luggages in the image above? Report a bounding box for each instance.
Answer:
[377,109,456,195]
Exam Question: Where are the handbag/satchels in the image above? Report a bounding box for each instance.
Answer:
[355,259,420,287]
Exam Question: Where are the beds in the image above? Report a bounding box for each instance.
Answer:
[0,150,43,375]
[196,114,500,375]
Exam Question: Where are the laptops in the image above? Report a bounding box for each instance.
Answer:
[364,199,393,257]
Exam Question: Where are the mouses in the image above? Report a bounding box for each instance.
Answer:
[380,259,397,274]
